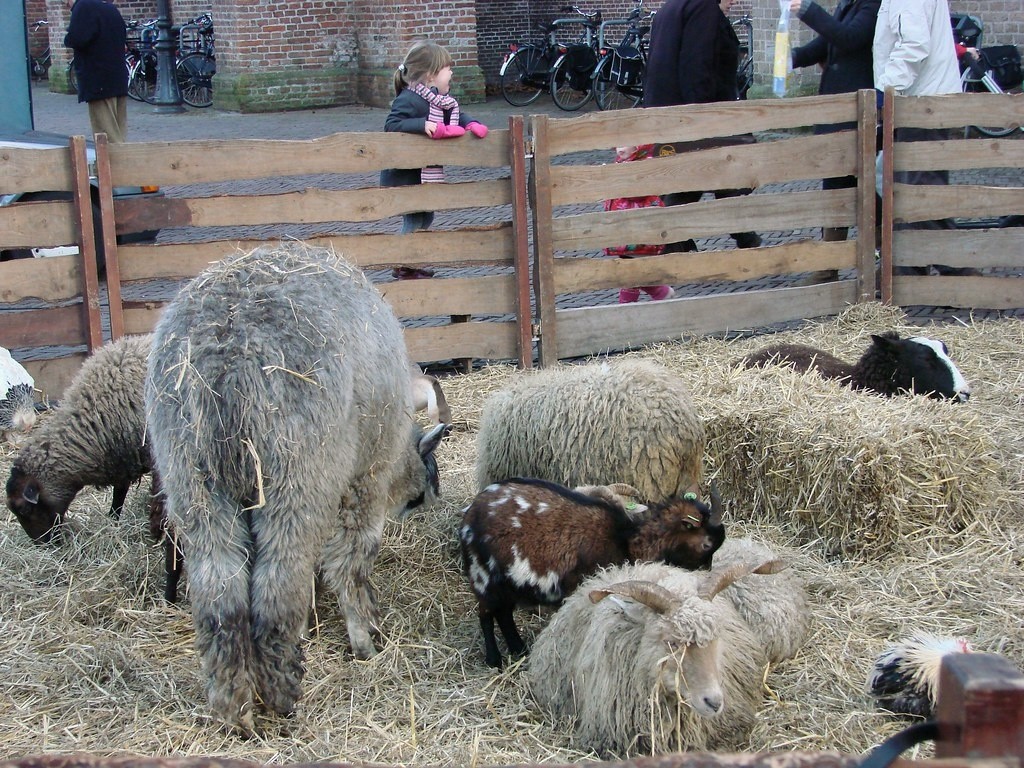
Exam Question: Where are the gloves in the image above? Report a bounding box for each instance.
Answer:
[465,122,488,138]
[431,122,465,139]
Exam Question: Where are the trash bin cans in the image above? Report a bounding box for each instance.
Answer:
[949,13,983,75]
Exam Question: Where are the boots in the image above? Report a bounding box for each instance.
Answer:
[875,224,898,291]
[788,228,848,288]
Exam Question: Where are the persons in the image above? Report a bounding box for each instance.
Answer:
[956,44,979,59]
[788,0,883,286]
[600,145,674,303]
[61,0,129,142]
[872,0,981,290]
[379,44,489,278]
[638,0,762,253]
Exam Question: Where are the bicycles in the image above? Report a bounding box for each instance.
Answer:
[29,20,50,81]
[499,0,753,111]
[65,12,216,108]
[953,27,1024,138]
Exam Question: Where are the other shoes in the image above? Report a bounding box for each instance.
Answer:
[662,286,674,300]
[392,263,434,281]
[745,234,767,248]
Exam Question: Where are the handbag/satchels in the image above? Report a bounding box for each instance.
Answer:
[652,134,757,196]
[603,43,645,88]
[549,41,598,91]
[974,46,1022,92]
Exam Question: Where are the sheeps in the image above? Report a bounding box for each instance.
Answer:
[0,244,1024,768]
